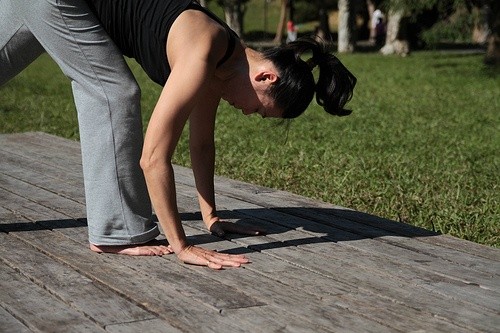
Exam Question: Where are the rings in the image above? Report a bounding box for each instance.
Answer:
[206,260,210,266]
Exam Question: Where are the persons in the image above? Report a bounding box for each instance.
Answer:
[286,20,299,42]
[371,5,384,29]
[0,0,356,268]
